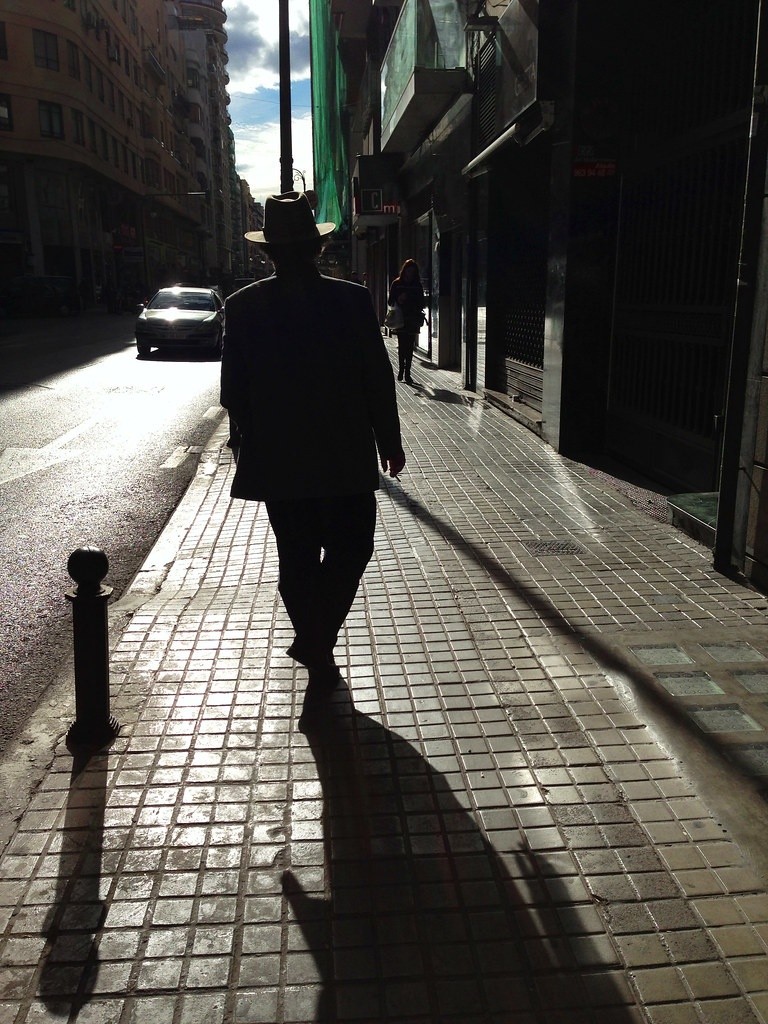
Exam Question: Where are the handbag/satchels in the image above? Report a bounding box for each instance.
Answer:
[383,301,404,329]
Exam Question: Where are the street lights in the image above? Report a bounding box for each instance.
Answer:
[138,189,223,295]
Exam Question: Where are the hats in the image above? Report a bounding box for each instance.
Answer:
[244,191,336,243]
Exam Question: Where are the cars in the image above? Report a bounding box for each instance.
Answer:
[134,281,225,358]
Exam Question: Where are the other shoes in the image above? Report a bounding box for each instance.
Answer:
[398,369,404,381]
[404,374,413,383]
[287,643,339,678]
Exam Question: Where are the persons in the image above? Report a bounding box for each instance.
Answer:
[218,192,410,687]
[388,258,424,385]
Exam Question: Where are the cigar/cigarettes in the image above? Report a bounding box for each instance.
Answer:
[388,467,402,483]
